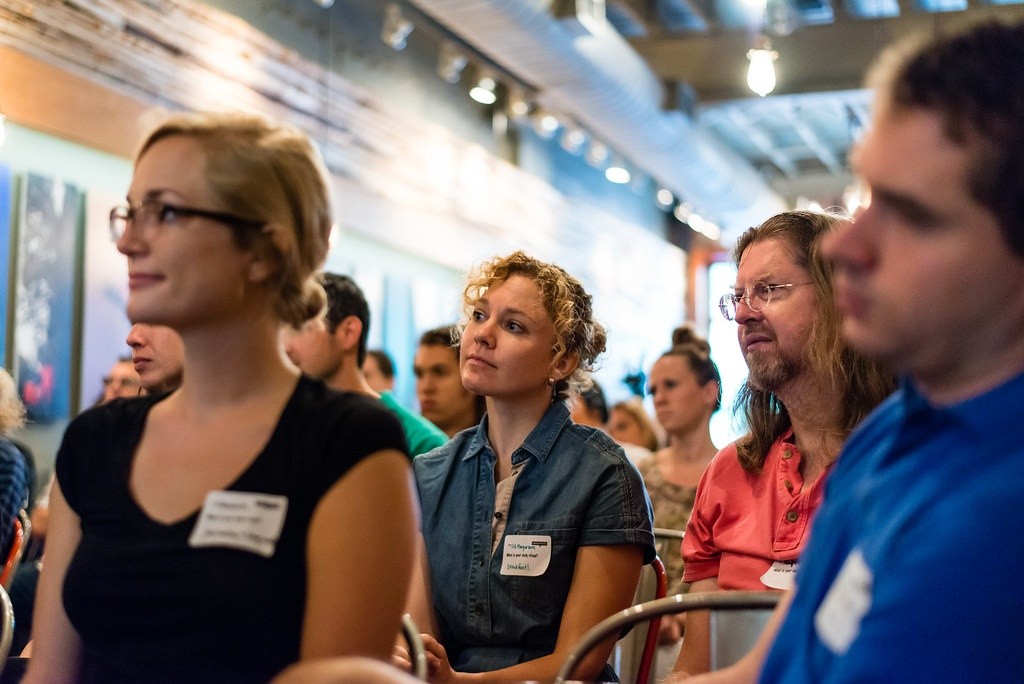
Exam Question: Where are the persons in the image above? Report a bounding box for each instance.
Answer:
[0,258,729,684]
[19,109,422,684]
[666,209,900,684]
[758,20,1024,684]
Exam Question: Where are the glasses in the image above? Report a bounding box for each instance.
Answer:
[717,277,819,321]
[107,198,268,246]
